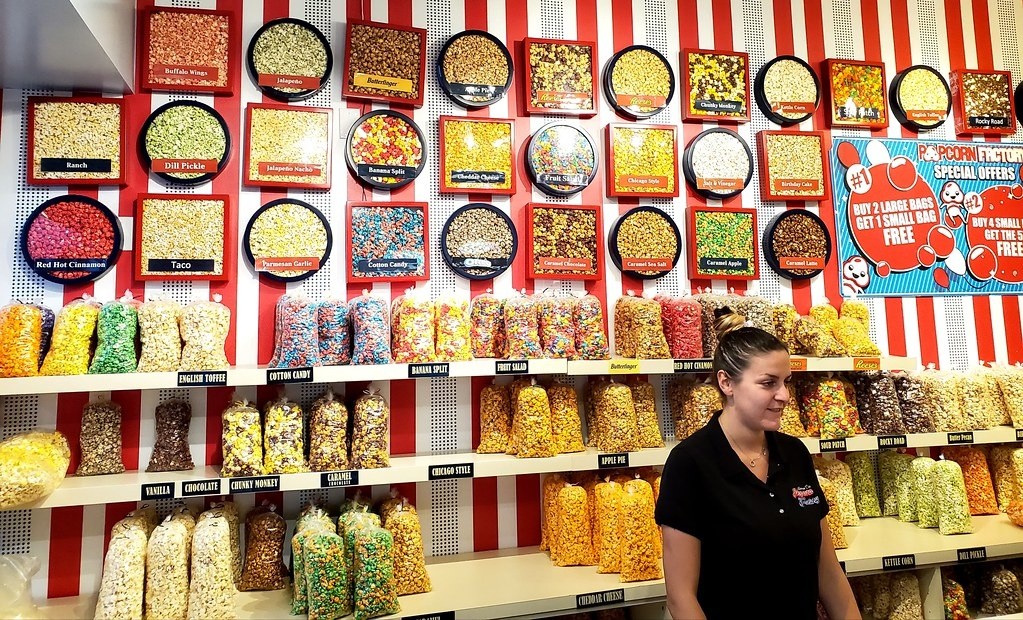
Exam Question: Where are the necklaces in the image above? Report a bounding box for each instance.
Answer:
[719,416,766,468]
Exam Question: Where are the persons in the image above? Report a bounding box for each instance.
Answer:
[655,306,862,620]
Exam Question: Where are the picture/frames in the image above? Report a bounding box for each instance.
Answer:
[440,202,519,282]
[142,3,235,98]
[341,16,429,110]
[524,202,604,283]
[27,94,129,189]
[606,119,681,201]
[607,205,683,281]
[436,28,516,108]
[436,111,517,197]
[245,17,335,101]
[519,35,600,118]
[526,120,600,197]
[22,193,125,288]
[682,49,1023,289]
[134,191,233,283]
[241,197,334,284]
[604,44,676,118]
[242,102,334,193]
[139,101,234,183]
[344,110,429,191]
[344,199,431,284]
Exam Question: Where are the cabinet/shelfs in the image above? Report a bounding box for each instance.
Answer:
[0,347,1023,620]
